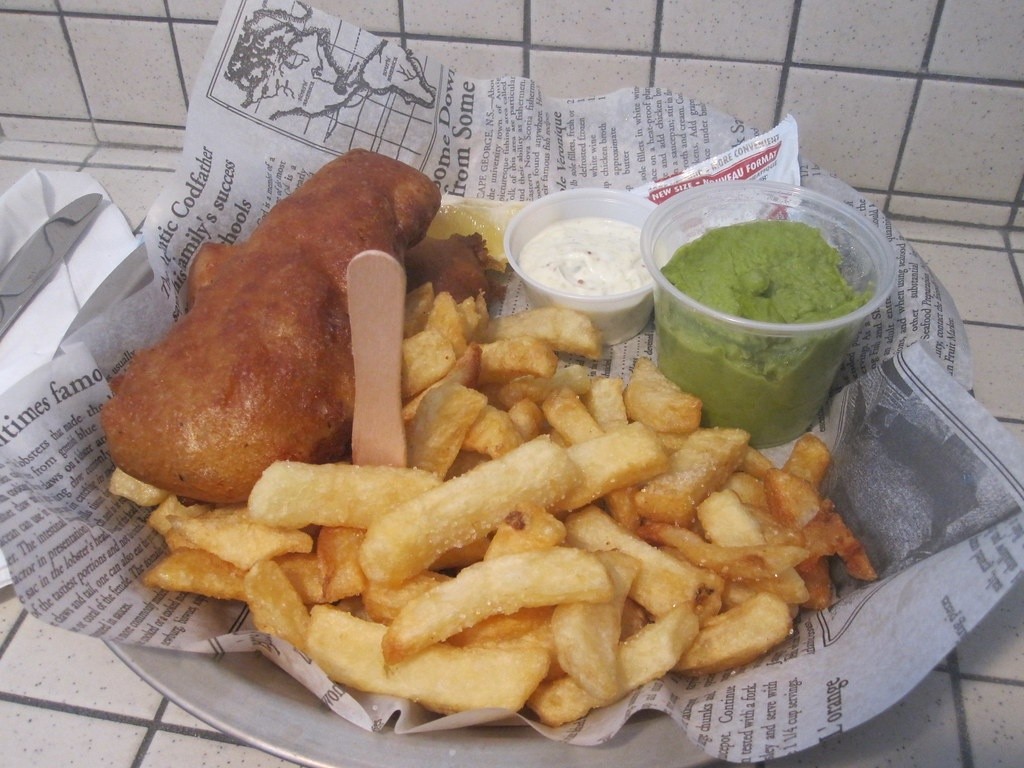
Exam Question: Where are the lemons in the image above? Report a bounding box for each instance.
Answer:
[422,192,536,262]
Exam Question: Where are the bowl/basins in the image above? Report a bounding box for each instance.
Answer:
[642,180,897,448]
[502,186,658,345]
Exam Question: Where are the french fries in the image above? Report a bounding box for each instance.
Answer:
[106,277,877,726]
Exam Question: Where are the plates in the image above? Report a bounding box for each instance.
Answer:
[86,160,980,763]
